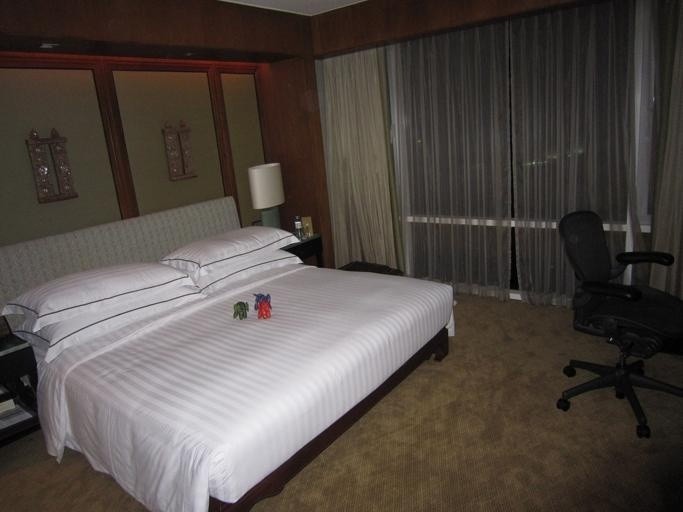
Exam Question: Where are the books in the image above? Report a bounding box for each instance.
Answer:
[0,389,34,430]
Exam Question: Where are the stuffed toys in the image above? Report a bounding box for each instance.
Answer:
[254,294,272,318]
[233,302,249,319]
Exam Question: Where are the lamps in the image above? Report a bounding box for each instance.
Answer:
[248,163,286,229]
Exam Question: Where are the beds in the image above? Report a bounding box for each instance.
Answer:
[0,194,454,512]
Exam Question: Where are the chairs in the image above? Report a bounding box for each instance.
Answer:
[556,209,682,438]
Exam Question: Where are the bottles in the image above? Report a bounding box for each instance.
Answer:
[293,215,303,240]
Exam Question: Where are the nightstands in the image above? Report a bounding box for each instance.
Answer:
[0,333,41,447]
[280,230,324,268]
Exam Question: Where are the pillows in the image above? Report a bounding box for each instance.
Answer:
[159,226,304,296]
[0,261,208,365]
